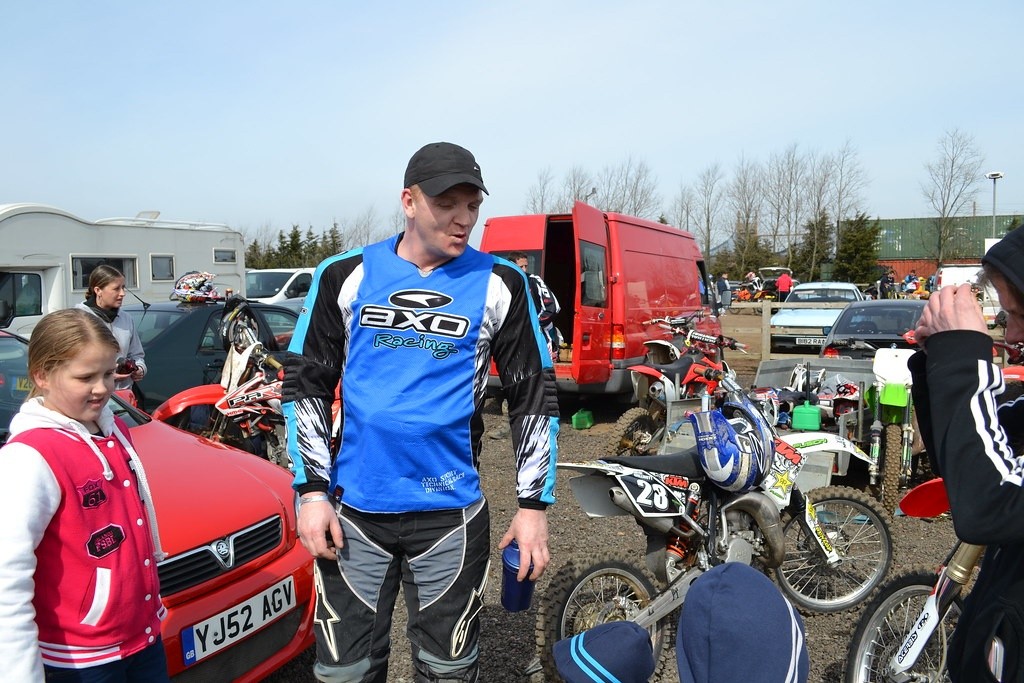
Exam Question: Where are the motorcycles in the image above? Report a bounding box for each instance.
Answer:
[534,366,897,683]
[839,540,987,683]
[604,309,749,456]
[728,289,773,316]
[860,343,922,520]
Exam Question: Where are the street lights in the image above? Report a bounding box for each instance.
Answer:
[984,171,1005,236]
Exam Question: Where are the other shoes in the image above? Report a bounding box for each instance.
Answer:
[488,422,510,439]
[480,400,503,416]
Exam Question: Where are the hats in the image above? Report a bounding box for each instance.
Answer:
[404,142,490,197]
[552,621,655,683]
[676,561,809,683]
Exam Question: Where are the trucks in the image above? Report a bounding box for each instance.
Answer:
[935,264,983,291]
[478,200,724,418]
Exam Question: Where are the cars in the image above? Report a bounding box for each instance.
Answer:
[819,299,929,361]
[0,298,301,428]
[246,266,316,324]
[770,282,865,355]
[1,327,317,683]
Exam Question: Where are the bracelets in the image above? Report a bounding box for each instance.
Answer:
[299,495,329,503]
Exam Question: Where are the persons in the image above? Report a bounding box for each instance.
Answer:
[74,265,147,406]
[775,271,792,302]
[908,224,1024,683]
[487,250,559,440]
[709,270,730,316]
[858,269,918,299]
[0,308,168,683]
[281,141,558,683]
[745,271,763,314]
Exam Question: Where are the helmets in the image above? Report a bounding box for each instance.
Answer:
[218,302,261,355]
[749,362,870,428]
[688,392,777,493]
[174,270,221,304]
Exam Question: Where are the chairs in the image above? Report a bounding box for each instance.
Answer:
[897,313,911,330]
[854,320,878,332]
